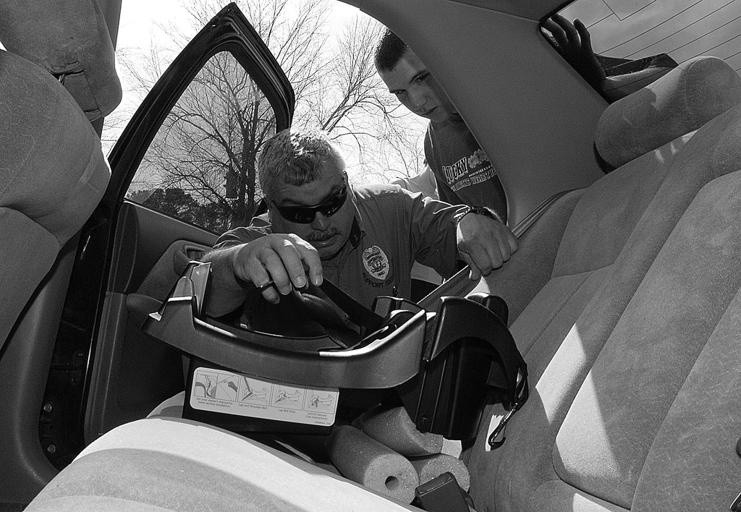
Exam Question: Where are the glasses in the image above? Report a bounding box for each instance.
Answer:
[266,185,347,224]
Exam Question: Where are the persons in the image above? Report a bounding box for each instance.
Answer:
[179,124,519,421]
[373,11,678,228]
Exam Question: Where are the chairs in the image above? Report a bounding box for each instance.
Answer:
[28,106,741,511]
[0,0,125,360]
[134,55,740,477]
